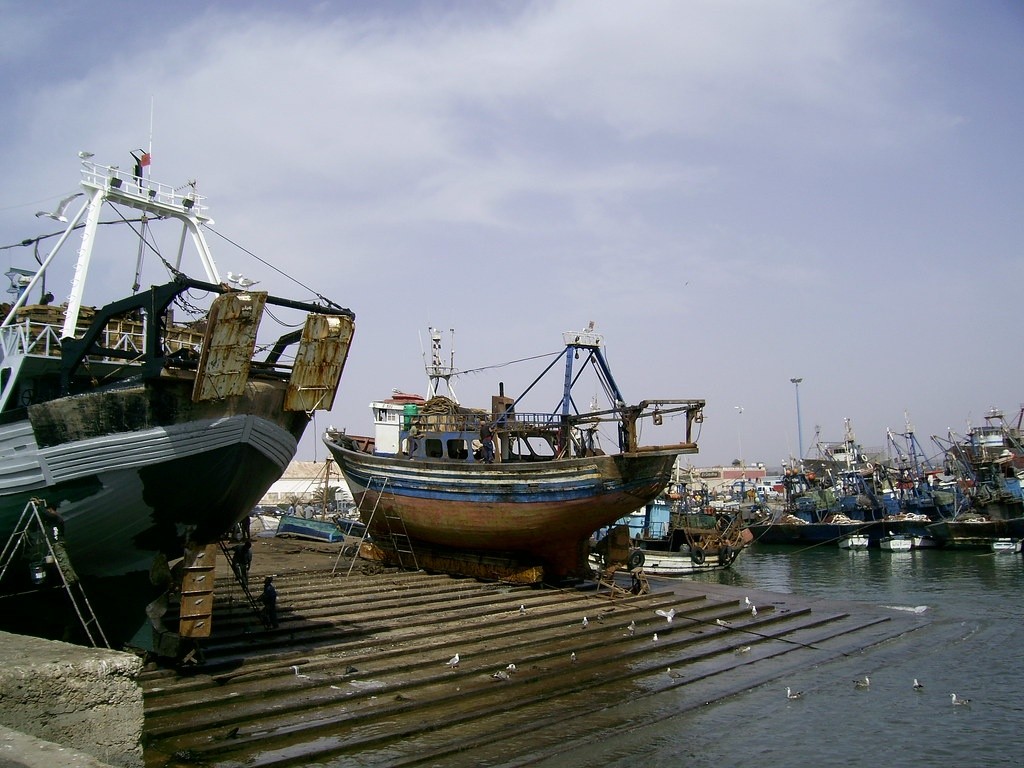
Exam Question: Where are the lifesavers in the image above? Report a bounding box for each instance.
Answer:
[690,547,706,565]
[720,547,728,560]
[627,549,645,571]
[728,548,733,557]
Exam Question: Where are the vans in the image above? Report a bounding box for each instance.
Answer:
[261,506,286,516]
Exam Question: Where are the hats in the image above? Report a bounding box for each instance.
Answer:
[480,421,485,425]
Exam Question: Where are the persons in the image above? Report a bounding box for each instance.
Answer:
[240,513,258,542]
[783,467,794,499]
[408,419,424,461]
[258,577,281,626]
[288,500,315,520]
[999,450,1023,497]
[695,477,761,503]
[223,541,253,590]
[480,420,496,464]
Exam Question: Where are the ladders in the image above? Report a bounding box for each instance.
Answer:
[0,498,111,650]
[331,475,420,579]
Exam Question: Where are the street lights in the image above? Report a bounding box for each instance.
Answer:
[788,375,803,467]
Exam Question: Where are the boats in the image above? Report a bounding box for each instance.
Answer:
[273,515,343,542]
[338,518,367,536]
[592,376,1024,575]
[321,321,706,584]
[0,107,357,652]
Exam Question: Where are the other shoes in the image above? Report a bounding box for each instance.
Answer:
[410,459,414,461]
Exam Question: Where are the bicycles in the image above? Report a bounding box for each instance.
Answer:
[343,537,368,561]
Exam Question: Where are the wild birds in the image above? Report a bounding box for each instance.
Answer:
[76,149,95,161]
[206,594,970,746]
[226,271,261,294]
[34,192,85,224]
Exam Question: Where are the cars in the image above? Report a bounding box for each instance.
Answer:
[249,507,264,517]
